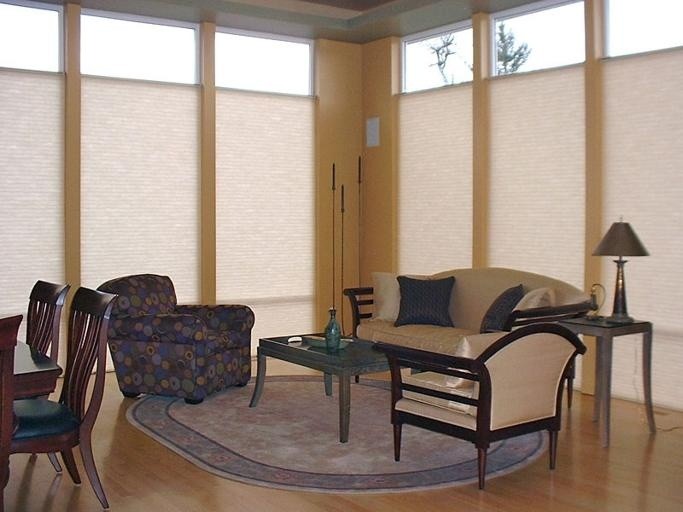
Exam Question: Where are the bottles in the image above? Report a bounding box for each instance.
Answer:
[326,308,341,353]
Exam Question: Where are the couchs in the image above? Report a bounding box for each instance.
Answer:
[344,268,590,408]
[95,274,255,404]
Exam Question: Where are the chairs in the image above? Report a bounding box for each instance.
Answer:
[14,288,118,510]
[26,281,72,364]
[371,324,586,489]
[343,287,374,382]
[1,314,24,493]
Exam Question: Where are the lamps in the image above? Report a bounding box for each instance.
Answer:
[592,222,650,324]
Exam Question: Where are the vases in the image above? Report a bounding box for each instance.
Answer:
[325,310,340,352]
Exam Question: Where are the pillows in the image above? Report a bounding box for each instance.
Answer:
[370,272,558,334]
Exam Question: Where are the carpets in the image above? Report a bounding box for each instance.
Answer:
[124,375,549,494]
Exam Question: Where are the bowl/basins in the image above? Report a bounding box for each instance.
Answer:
[302,335,355,350]
